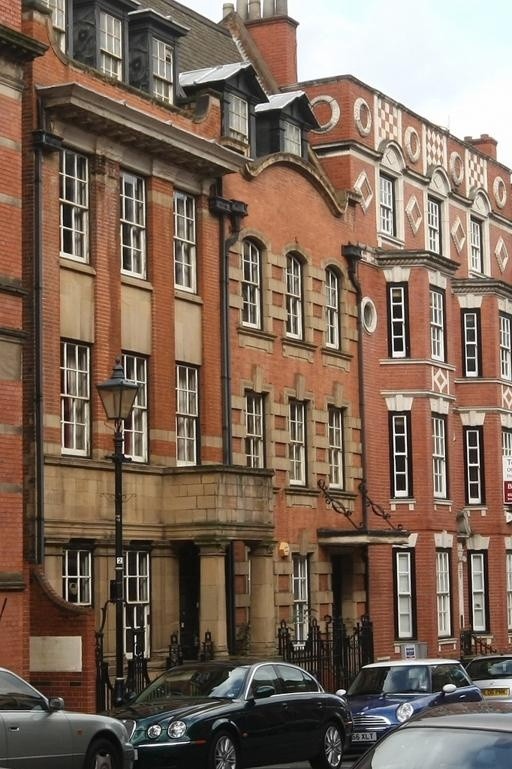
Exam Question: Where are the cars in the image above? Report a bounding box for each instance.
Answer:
[101,655,353,769]
[0,666,135,769]
[338,657,484,744]
[466,652,512,713]
[353,697,512,768]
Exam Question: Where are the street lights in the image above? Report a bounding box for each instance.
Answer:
[97,357,140,704]
